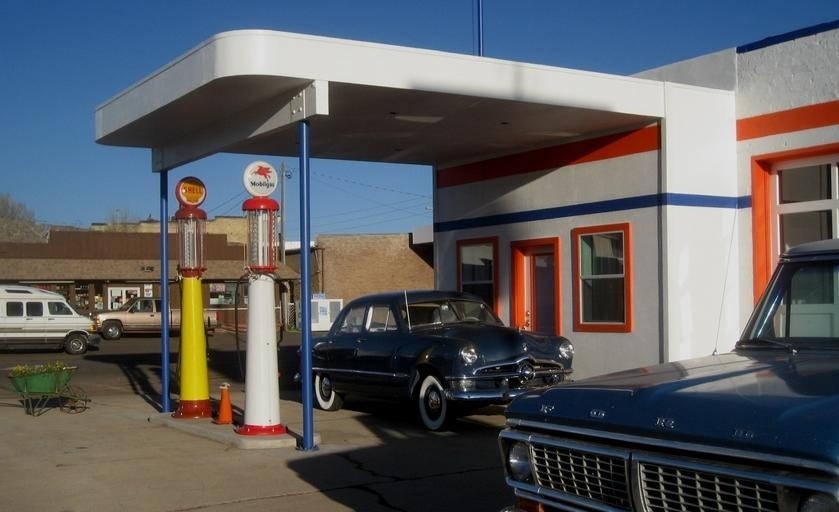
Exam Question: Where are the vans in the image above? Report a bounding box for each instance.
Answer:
[0,284,102,355]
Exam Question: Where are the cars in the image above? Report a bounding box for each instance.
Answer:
[293,290,575,432]
[498,237,839,512]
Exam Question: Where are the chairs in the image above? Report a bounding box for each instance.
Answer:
[433,303,458,324]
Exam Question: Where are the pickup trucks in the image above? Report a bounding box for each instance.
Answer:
[95,297,219,340]
